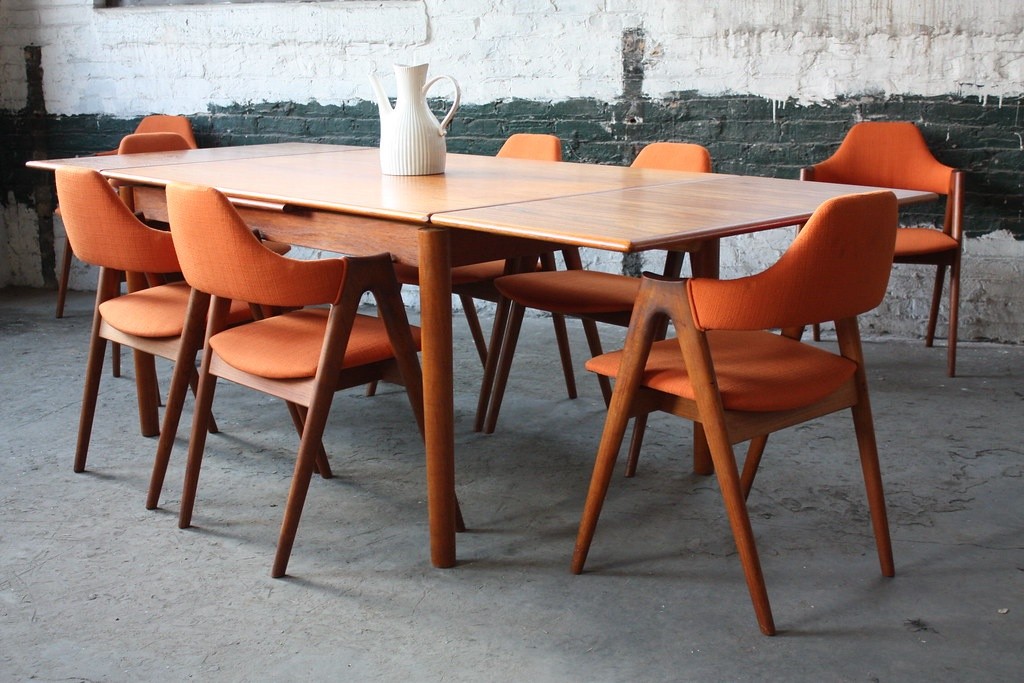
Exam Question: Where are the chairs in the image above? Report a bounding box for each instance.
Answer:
[109,133,292,434]
[570,193,897,637]
[364,135,577,433]
[166,177,465,577]
[790,123,963,378]
[482,144,710,479]
[55,114,198,317]
[54,166,331,512]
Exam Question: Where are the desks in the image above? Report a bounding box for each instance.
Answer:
[26,141,941,568]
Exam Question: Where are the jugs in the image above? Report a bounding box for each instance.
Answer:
[368,63,460,175]
